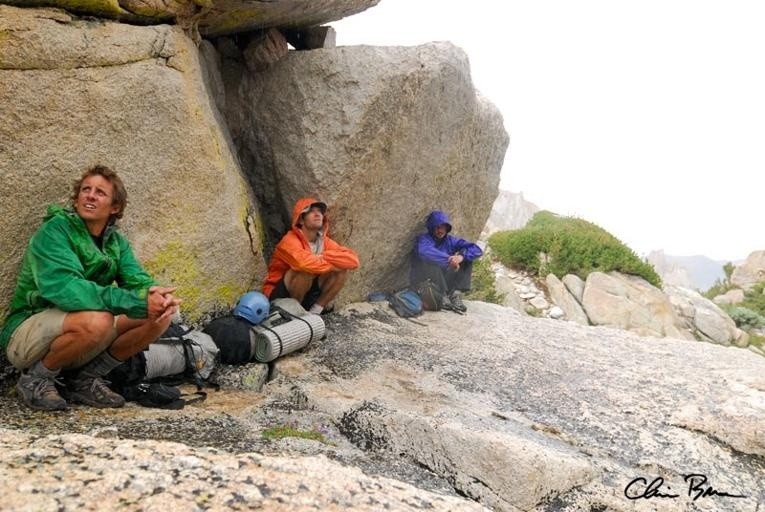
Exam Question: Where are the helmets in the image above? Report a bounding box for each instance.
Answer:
[232,291,271,325]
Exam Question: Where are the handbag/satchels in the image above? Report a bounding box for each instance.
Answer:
[389,287,423,317]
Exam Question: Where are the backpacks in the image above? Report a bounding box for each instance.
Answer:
[118,326,217,387]
[201,296,315,367]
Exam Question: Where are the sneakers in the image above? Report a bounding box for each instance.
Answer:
[62,368,126,409]
[440,295,454,311]
[448,292,468,313]
[13,369,67,412]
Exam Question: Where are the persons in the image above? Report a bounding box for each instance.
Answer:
[409,211,482,315]
[262,196,359,316]
[0,161,182,413]
